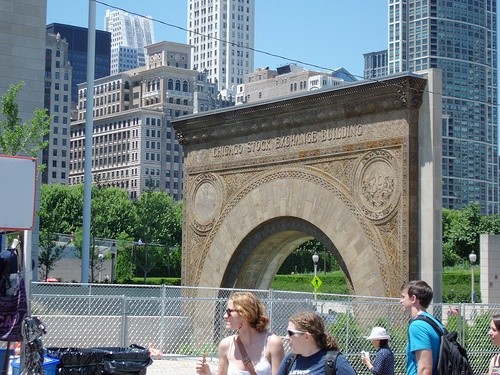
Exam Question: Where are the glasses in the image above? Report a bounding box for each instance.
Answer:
[224,308,241,316]
[287,329,311,337]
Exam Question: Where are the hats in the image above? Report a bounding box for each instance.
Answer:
[365,326,390,340]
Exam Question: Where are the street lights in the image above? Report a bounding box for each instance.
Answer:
[469,251,476,304]
[98,253,104,283]
[311,251,319,301]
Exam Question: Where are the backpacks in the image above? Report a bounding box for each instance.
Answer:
[408,314,474,375]
[0,248,18,297]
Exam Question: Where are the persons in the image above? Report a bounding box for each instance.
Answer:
[400,280,449,375]
[196,292,285,375]
[447,307,459,318]
[360,327,395,375]
[487,314,500,375]
[275,311,357,375]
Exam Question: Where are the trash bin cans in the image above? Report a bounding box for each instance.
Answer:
[0,348,13,375]
[43,347,103,375]
[90,345,150,375]
[11,357,60,375]
